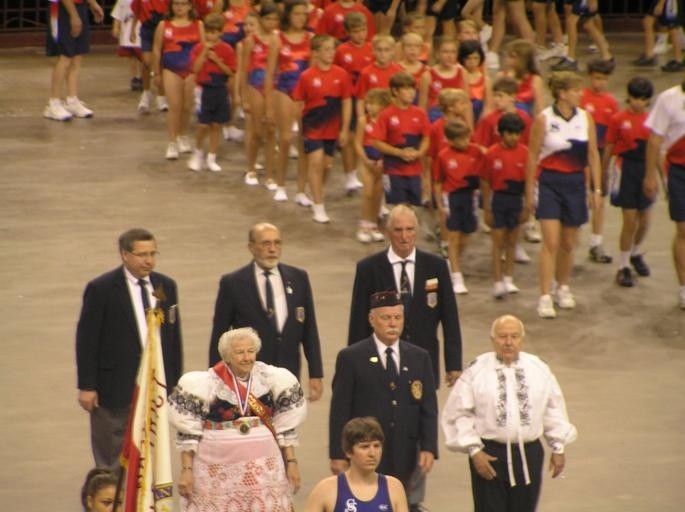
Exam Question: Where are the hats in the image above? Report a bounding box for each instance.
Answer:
[371,290,402,308]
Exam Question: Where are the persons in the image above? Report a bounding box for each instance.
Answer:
[347,203,463,387]
[440,314,579,511]
[80,468,125,512]
[208,222,324,403]
[328,291,439,512]
[300,415,412,511]
[76,228,184,467]
[167,327,308,512]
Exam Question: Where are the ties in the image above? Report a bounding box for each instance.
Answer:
[138,278,152,325]
[261,270,277,329]
[399,261,412,316]
[384,348,400,387]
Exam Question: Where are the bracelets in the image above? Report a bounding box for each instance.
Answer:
[181,464,193,469]
[286,457,297,463]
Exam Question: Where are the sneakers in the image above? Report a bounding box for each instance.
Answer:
[630,253,650,277]
[187,152,202,170]
[588,57,617,75]
[492,280,505,297]
[540,46,552,61]
[677,285,685,309]
[481,221,490,233]
[503,275,520,293]
[176,135,192,153]
[356,223,371,242]
[272,186,289,202]
[550,34,570,46]
[42,96,73,120]
[550,56,579,73]
[555,46,570,55]
[524,222,541,244]
[514,245,530,264]
[660,59,684,73]
[483,49,501,69]
[371,222,386,241]
[555,285,575,308]
[138,90,151,115]
[342,173,356,190]
[205,156,222,172]
[244,171,260,186]
[654,33,668,54]
[588,245,612,266]
[354,176,364,187]
[222,125,230,140]
[229,125,245,142]
[377,204,391,221]
[482,24,492,41]
[616,268,635,287]
[261,178,278,191]
[65,96,94,119]
[130,76,143,91]
[294,191,314,207]
[155,96,168,110]
[253,160,263,170]
[632,52,657,66]
[536,294,556,318]
[288,144,300,159]
[164,142,179,159]
[584,43,600,54]
[312,204,331,223]
[450,270,470,294]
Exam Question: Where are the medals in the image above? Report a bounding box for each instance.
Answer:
[223,362,255,436]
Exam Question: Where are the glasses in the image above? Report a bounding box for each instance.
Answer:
[126,247,162,260]
[252,237,283,249]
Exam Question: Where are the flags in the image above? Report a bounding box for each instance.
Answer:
[119,305,174,512]
